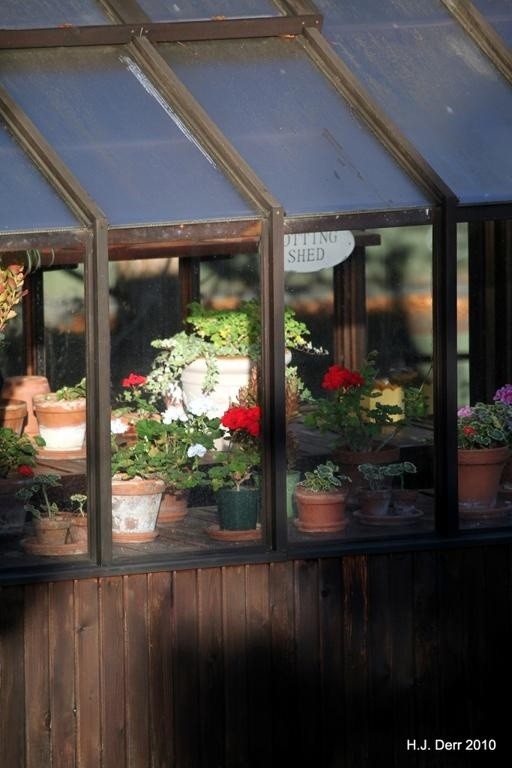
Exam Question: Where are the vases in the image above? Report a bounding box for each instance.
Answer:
[1,446,511,562]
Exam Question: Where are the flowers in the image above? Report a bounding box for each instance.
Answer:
[0,263,512,521]
